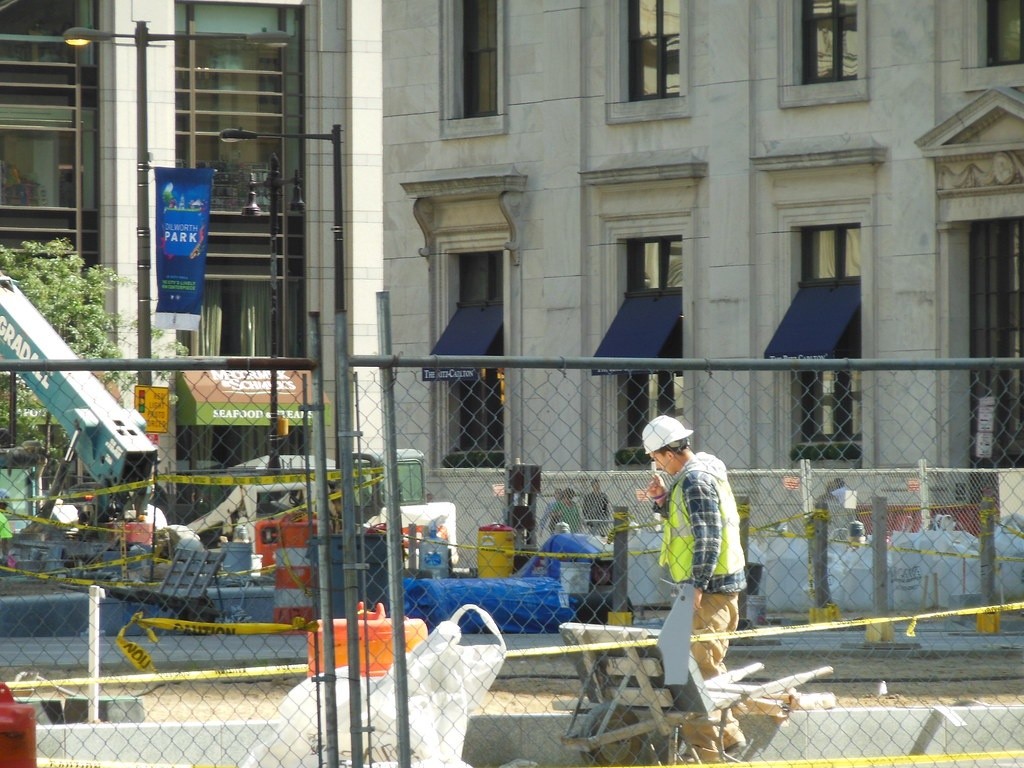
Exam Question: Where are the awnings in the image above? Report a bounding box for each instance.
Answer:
[422,304,501,380]
[592,290,680,375]
[764,280,857,361]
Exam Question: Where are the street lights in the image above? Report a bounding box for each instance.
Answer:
[220,123,364,768]
[62,19,293,434]
[241,153,306,475]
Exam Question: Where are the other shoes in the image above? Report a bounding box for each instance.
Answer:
[723,740,747,758]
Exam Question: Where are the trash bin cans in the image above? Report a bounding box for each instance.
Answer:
[479,524,515,579]
[307,533,391,618]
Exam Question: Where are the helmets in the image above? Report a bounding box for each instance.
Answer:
[642,416,694,454]
[0,488,10,505]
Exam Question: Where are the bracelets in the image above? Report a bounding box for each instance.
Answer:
[650,493,667,503]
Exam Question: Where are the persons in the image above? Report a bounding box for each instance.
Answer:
[642,413,751,763]
[0,489,12,538]
[538,480,611,536]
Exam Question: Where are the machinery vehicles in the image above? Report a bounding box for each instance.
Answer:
[350,446,460,579]
[0,271,277,638]
